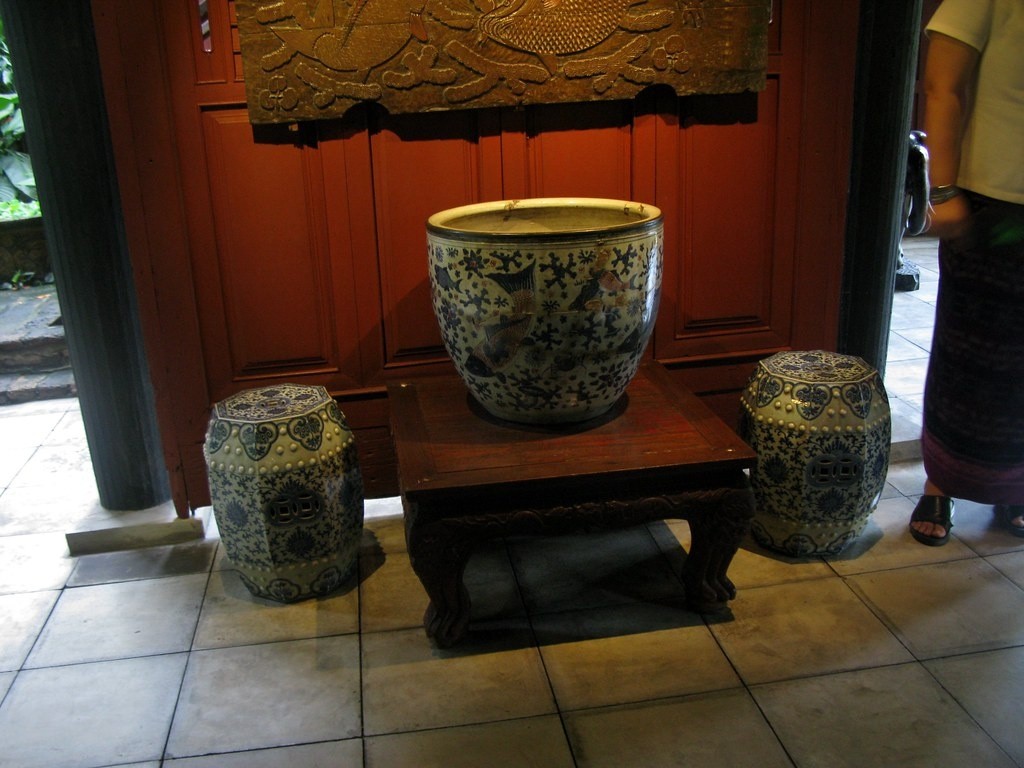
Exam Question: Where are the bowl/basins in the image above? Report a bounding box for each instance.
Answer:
[425,197,664,422]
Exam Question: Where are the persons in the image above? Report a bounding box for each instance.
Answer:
[909,0,1024,547]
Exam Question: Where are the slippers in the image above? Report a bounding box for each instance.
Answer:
[997,498,1024,537]
[908,494,954,547]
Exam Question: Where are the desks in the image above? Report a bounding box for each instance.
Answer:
[380,361,757,652]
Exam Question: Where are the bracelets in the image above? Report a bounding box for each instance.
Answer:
[929,183,961,206]
